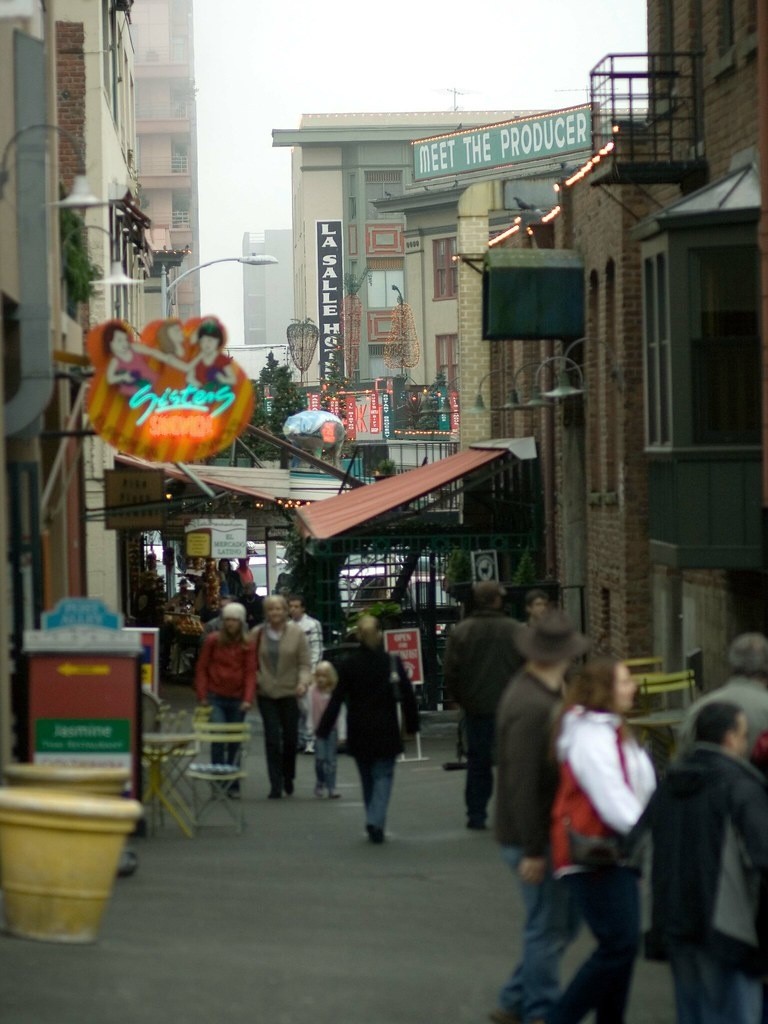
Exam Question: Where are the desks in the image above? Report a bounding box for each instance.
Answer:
[140,732,198,834]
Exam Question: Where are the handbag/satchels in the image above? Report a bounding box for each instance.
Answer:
[389,655,401,701]
[566,831,617,866]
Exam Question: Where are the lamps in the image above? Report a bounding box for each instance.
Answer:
[418,337,586,416]
[2,124,109,210]
[61,225,147,285]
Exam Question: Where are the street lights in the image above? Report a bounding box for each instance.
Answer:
[160,255,280,318]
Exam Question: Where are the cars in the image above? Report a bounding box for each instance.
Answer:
[146,538,291,598]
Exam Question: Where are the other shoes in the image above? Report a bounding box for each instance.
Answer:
[268,790,283,800]
[314,786,323,798]
[489,1008,522,1024]
[329,790,341,799]
[466,819,487,829]
[642,940,667,962]
[227,784,242,799]
[365,823,384,842]
[283,780,294,794]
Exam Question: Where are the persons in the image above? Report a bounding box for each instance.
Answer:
[248,596,313,798]
[195,599,255,799]
[443,583,657,1024]
[238,583,264,626]
[646,706,768,1024]
[678,630,768,763]
[301,662,347,797]
[288,597,323,753]
[176,557,253,599]
[315,615,421,840]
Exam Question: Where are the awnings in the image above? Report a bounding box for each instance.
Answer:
[118,453,275,504]
[294,438,537,538]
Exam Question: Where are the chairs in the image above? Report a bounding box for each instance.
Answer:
[184,721,253,831]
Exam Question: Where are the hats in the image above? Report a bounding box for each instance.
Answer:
[223,602,246,619]
[513,609,589,666]
[476,579,506,603]
